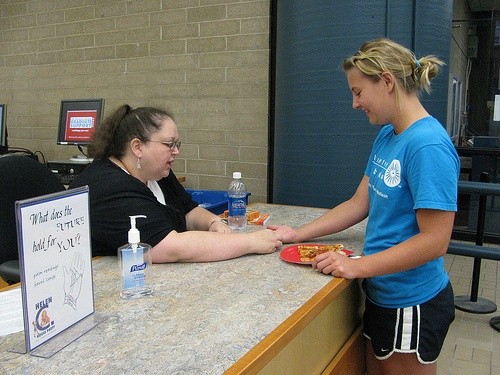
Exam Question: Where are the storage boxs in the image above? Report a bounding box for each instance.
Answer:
[185,189,251,215]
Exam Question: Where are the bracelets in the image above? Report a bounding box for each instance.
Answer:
[210,219,229,225]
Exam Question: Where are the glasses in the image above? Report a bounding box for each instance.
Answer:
[143,140,182,151]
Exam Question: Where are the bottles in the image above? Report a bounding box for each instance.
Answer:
[227,172,247,230]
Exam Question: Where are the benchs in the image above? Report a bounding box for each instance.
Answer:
[446,242,500,331]
[454,181,500,314]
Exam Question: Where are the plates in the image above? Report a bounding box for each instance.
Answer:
[280,243,355,264]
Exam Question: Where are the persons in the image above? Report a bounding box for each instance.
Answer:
[266,38,460,375]
[66,105,283,263]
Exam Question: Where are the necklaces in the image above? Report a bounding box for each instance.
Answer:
[117,158,131,174]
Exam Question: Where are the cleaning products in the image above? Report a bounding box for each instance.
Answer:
[118,214,153,299]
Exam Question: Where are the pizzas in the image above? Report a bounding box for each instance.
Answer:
[297,244,345,262]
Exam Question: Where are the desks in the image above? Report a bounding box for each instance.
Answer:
[0,202,368,375]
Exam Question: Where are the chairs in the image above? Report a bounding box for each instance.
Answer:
[0,152,67,285]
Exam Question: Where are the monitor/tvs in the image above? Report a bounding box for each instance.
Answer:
[55,98,105,159]
[0,103,10,152]
